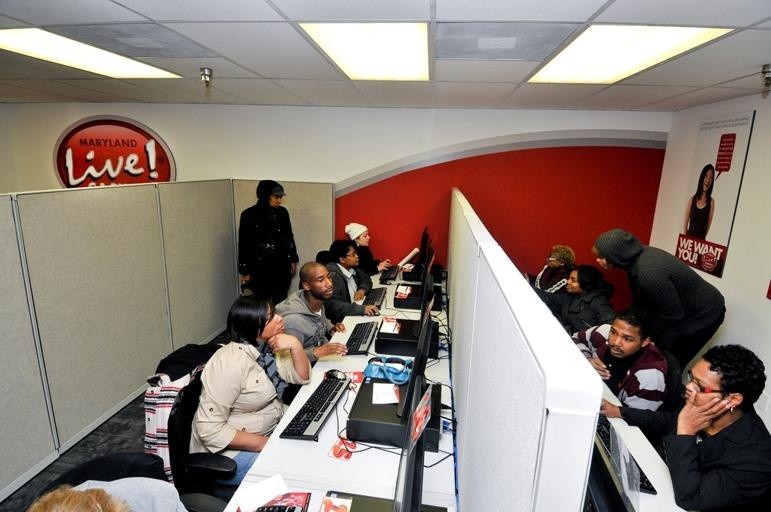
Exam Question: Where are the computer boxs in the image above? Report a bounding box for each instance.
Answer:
[373,316,440,360]
[319,490,448,512]
[392,282,444,312]
[345,376,441,453]
[402,263,444,283]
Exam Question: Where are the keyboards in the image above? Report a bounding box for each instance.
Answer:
[343,321,378,356]
[597,412,658,495]
[254,506,300,512]
[379,265,399,281]
[278,375,352,441]
[361,286,387,309]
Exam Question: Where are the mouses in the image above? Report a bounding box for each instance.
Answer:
[378,280,392,285]
[370,310,381,316]
[325,368,348,380]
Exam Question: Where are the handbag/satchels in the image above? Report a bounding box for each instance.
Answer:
[364,357,414,385]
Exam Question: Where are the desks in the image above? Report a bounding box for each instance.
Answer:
[224,269,451,511]
[601,382,696,512]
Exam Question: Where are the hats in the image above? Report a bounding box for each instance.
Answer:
[273,188,286,196]
[344,222,368,241]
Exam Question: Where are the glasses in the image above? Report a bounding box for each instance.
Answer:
[546,257,561,262]
[686,369,723,395]
[345,251,358,258]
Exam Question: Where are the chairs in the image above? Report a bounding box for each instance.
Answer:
[37,453,169,482]
[170,379,238,502]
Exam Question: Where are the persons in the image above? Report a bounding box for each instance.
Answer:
[345,223,391,277]
[274,262,348,364]
[684,163,715,241]
[524,263,616,337]
[528,245,575,294]
[593,227,727,373]
[189,294,311,487]
[237,180,299,305]
[24,476,188,512]
[598,344,771,512]
[323,239,378,325]
[572,305,668,412]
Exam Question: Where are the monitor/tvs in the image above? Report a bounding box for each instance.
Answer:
[395,309,433,418]
[419,274,434,324]
[392,375,427,512]
[421,237,433,274]
[418,225,430,245]
